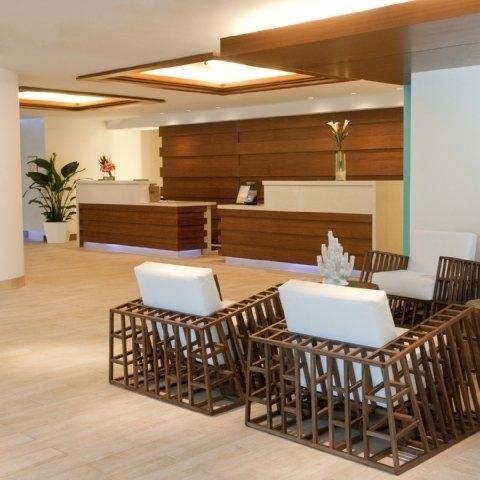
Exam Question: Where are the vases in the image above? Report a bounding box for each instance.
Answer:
[334,152,347,181]
[101,168,116,181]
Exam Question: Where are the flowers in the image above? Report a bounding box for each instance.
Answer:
[322,119,352,171]
[99,153,118,177]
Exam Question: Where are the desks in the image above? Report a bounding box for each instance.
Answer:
[306,277,382,303]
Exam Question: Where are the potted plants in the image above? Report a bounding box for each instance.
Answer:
[22,153,86,243]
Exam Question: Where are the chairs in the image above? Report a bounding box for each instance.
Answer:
[358,229,479,323]
[242,277,475,475]
[109,258,284,419]
